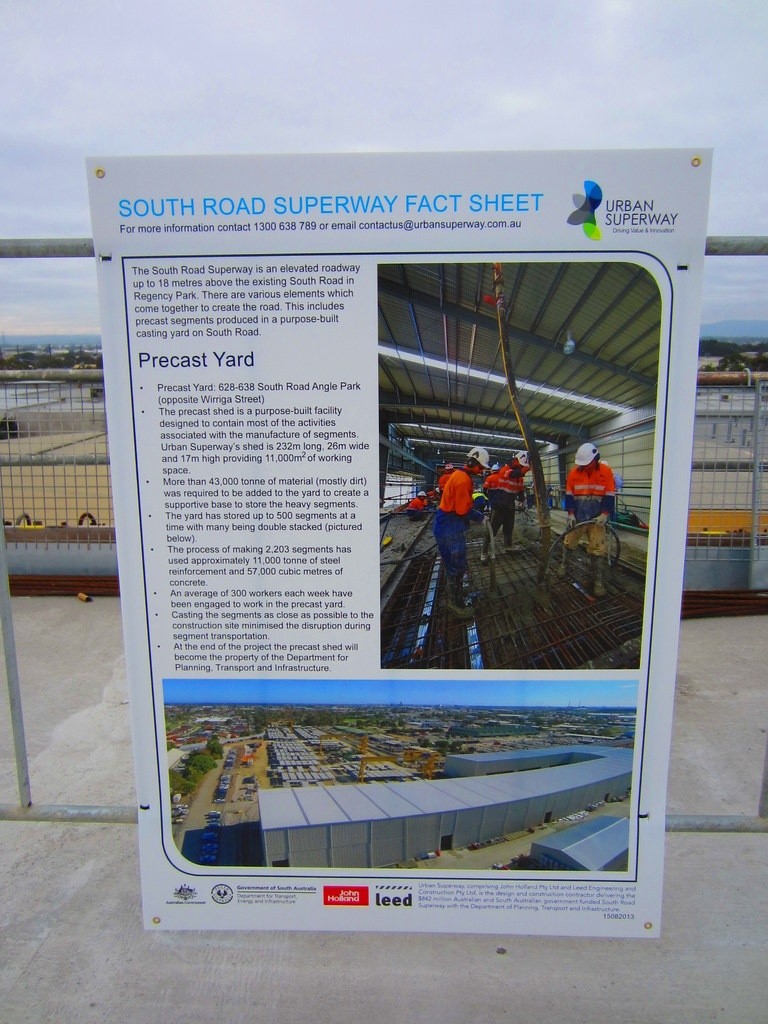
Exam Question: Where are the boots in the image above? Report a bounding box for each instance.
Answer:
[480,535,491,562]
[557,545,572,578]
[593,555,606,596]
[447,581,474,619]
[503,533,519,552]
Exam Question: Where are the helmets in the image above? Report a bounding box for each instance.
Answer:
[516,452,530,468]
[491,464,500,471]
[445,464,453,469]
[418,491,427,497]
[436,488,439,492]
[467,447,491,469]
[575,443,599,466]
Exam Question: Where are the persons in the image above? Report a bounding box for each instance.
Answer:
[557,443,616,598]
[433,448,490,619]
[439,464,454,502]
[601,461,623,531]
[472,493,488,514]
[481,451,531,563]
[427,487,440,511]
[406,491,428,521]
